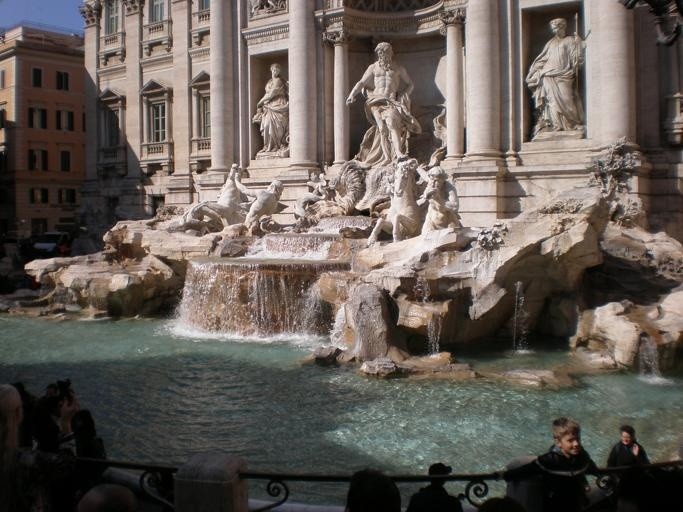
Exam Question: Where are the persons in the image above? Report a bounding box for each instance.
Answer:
[526,18,585,137]
[344,469,400,509]
[0,382,45,510]
[76,485,137,511]
[67,411,101,470]
[235,173,284,227]
[54,232,70,257]
[252,63,289,151]
[495,419,597,510]
[14,379,77,454]
[421,166,459,235]
[406,463,462,511]
[608,424,650,467]
[346,42,414,164]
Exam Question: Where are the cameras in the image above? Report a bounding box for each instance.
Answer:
[45,379,73,408]
